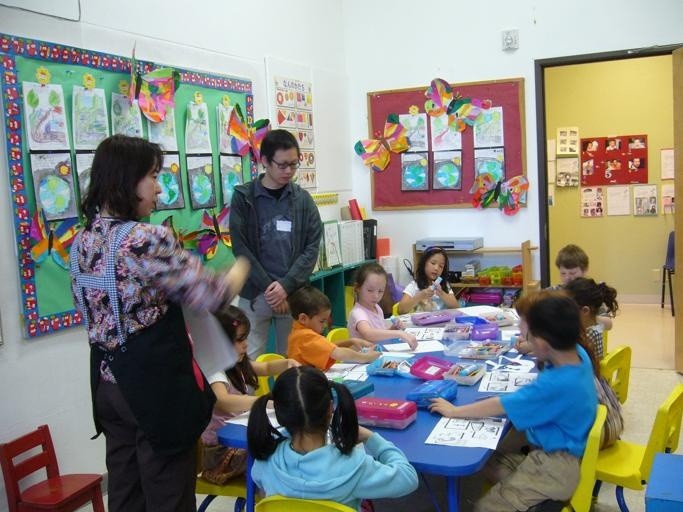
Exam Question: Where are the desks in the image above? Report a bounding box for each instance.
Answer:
[216,312,540,512]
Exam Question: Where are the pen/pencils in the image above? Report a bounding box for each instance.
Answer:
[376,360,400,376]
[451,367,480,386]
[445,327,470,342]
[459,343,504,359]
[419,315,448,319]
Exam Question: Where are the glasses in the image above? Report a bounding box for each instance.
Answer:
[270,157,302,169]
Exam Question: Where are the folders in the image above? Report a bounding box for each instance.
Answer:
[363,219,377,259]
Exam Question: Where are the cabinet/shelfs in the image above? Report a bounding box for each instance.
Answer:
[411,239,542,308]
[309,257,378,327]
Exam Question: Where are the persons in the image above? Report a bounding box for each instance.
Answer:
[284,285,383,373]
[514,289,600,377]
[196,304,303,488]
[586,141,593,149]
[395,245,459,315]
[633,138,641,148]
[513,276,619,360]
[631,156,641,168]
[585,161,591,174]
[606,140,616,150]
[227,127,324,363]
[538,245,613,330]
[65,132,254,512]
[346,262,418,351]
[612,159,619,168]
[243,363,421,511]
[424,278,620,512]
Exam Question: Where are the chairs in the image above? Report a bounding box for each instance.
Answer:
[392,302,414,317]
[193,437,248,512]
[252,352,287,396]
[558,403,608,512]
[659,230,675,317]
[0,425,105,512]
[325,327,349,364]
[255,495,359,512]
[592,382,683,512]
[598,330,632,405]
